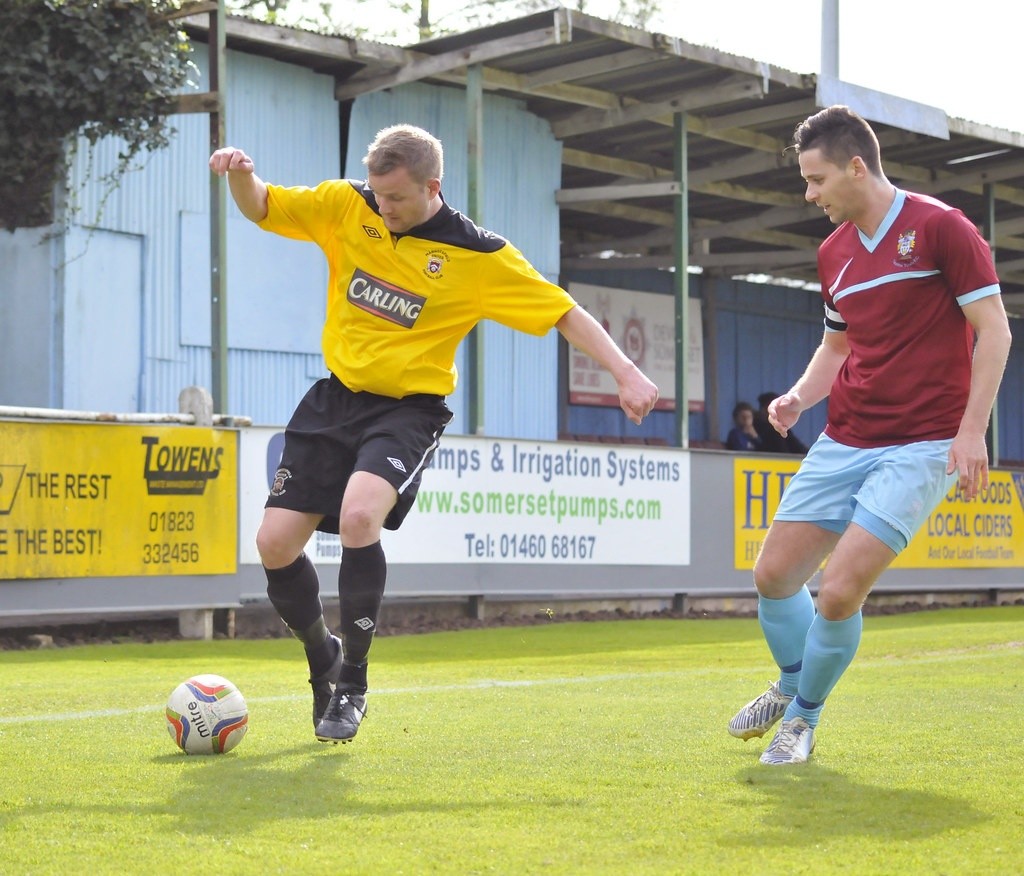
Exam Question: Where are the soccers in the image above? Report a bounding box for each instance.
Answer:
[164,673,253,755]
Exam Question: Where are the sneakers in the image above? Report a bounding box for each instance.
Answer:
[728,679,795,741]
[316,687,368,745]
[758,717,816,765]
[308,635,343,728]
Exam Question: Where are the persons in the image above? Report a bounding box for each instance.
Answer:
[754,393,808,453]
[726,403,768,451]
[728,103,1012,766]
[208,124,659,746]
